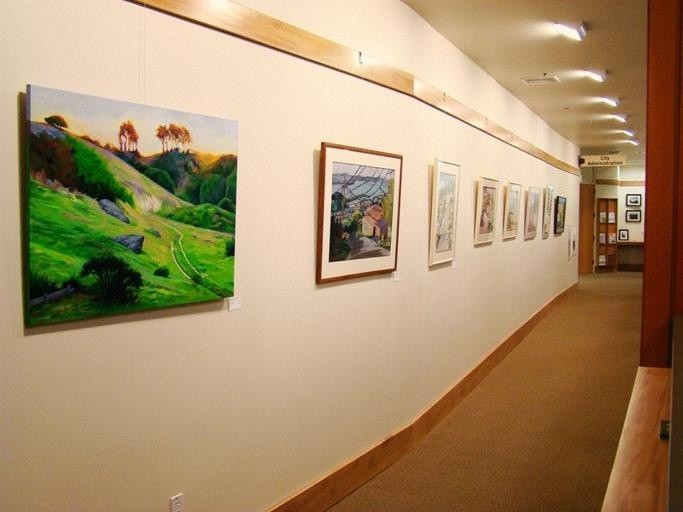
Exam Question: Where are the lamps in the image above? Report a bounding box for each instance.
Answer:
[555,18,641,148]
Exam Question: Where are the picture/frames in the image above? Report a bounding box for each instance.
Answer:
[625,193,641,206]
[472,173,566,253]
[618,229,628,240]
[311,140,403,284]
[425,158,458,269]
[624,210,641,222]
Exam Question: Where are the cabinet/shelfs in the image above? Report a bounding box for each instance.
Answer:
[596,198,617,273]
